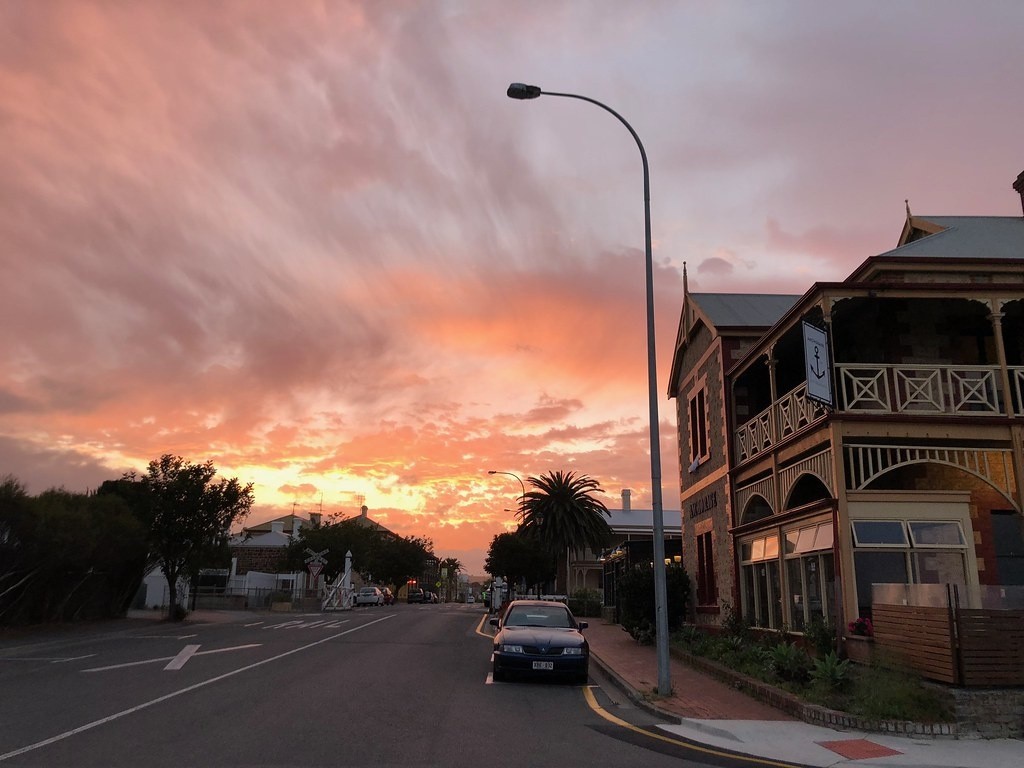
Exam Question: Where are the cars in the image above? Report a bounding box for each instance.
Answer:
[379,586,395,606]
[489,599,590,686]
[467,596,475,603]
[356,586,386,606]
[407,587,439,604]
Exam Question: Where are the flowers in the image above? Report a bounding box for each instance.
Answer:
[847,618,873,636]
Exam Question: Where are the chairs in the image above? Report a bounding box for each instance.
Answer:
[544,614,567,626]
[511,613,528,625]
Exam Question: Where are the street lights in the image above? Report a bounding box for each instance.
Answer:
[488,470,526,529]
[506,83,672,697]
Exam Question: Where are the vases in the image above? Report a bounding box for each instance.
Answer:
[845,634,875,665]
